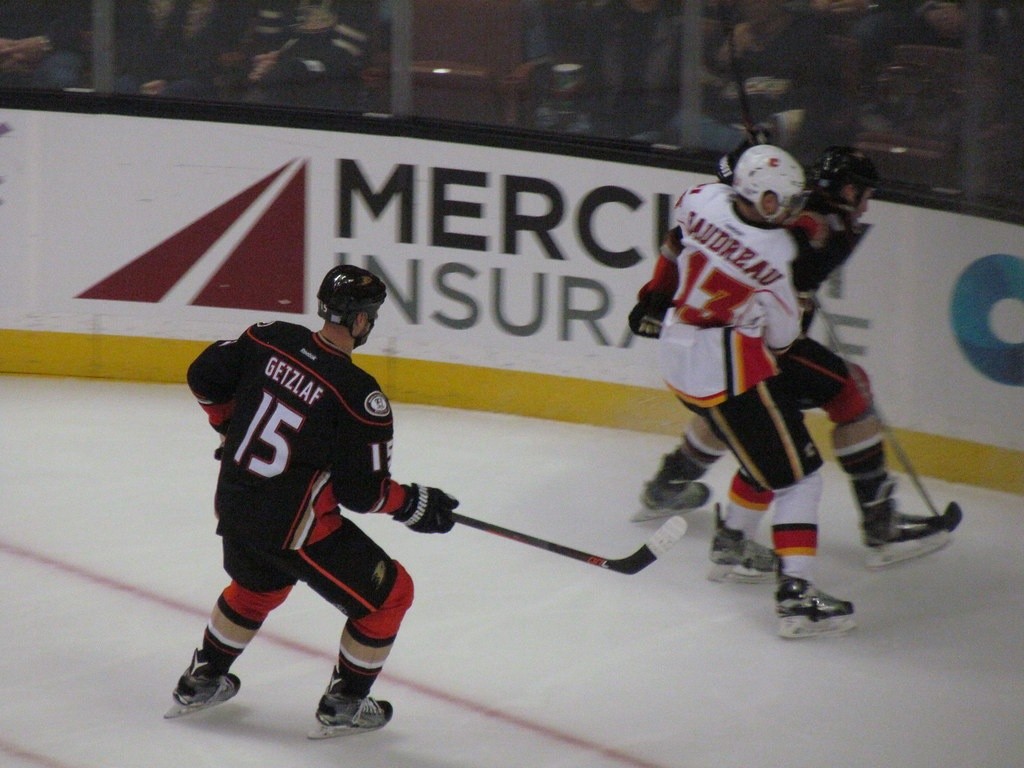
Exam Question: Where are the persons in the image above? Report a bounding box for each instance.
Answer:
[0,0,389,115]
[512,1,1024,222]
[164,265,460,740]
[620,129,949,638]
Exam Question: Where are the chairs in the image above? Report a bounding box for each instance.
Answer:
[366,0,532,128]
[853,42,969,184]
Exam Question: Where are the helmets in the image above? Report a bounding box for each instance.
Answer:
[316,265,386,348]
[809,145,875,205]
[731,144,805,221]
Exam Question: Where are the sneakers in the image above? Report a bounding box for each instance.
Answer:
[307,666,393,739]
[705,501,780,585]
[629,455,709,523]
[859,481,950,566]
[163,649,242,719]
[774,558,856,638]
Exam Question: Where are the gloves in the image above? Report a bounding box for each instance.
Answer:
[718,152,742,185]
[387,482,459,534]
[628,280,674,339]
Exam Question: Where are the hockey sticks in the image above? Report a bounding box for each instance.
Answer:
[811,292,962,532]
[453,512,688,575]
